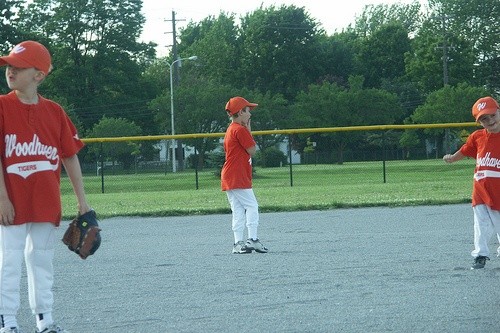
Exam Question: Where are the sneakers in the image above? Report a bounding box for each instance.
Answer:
[0,321,66,333]
[234,238,268,254]
[471,256,486,269]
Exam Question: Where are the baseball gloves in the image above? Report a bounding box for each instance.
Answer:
[61,209,103,260]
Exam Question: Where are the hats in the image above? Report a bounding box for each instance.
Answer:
[0,41,51,74]
[225,97,260,116]
[471,96,500,124]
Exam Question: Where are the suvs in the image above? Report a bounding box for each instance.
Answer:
[97,154,119,176]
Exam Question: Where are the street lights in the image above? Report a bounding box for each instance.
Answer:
[144,56,198,173]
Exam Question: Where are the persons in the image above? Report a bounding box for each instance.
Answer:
[221,96,268,254]
[443,97,500,270]
[0,40,101,333]
